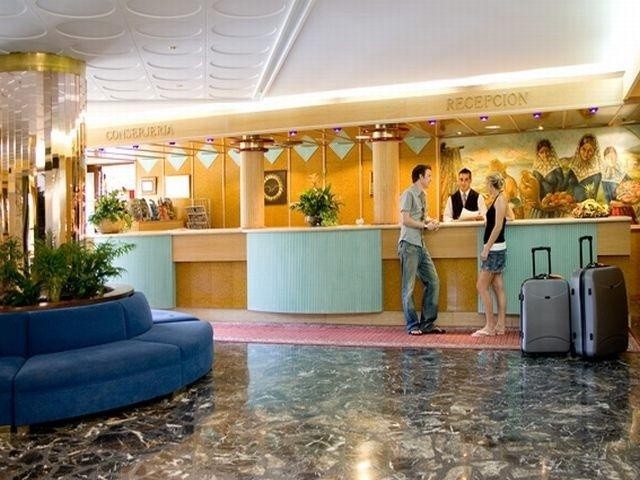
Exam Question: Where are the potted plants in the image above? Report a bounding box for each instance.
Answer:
[88,189,132,234]
[288,182,340,227]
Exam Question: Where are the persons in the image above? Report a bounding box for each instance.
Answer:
[397,164,446,335]
[442,167,487,223]
[526,132,632,219]
[470,172,515,337]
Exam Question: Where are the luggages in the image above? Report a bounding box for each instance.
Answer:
[569,261,629,361]
[518,274,573,355]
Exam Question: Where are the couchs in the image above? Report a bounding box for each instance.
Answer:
[0,289,213,434]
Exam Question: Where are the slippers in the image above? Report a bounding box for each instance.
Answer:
[410,328,424,336]
[431,326,445,334]
[470,328,496,338]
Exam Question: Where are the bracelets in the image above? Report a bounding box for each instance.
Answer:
[424,223,427,230]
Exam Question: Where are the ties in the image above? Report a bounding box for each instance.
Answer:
[462,193,467,206]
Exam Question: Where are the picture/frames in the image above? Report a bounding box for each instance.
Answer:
[140,176,157,195]
